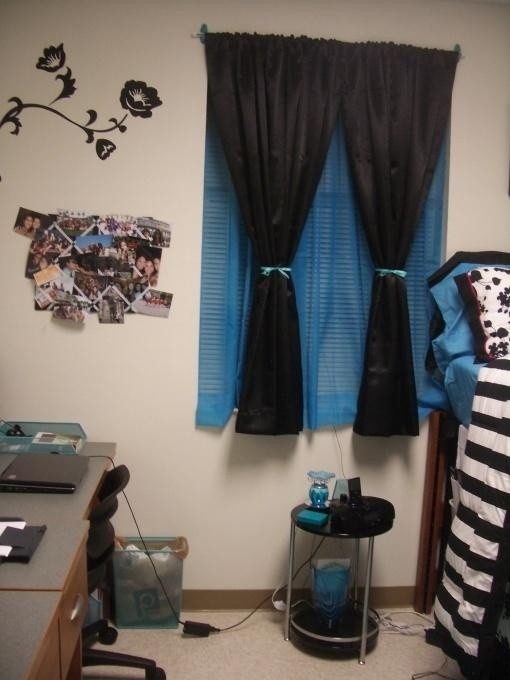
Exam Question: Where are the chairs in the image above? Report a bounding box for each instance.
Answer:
[72,464,166,680]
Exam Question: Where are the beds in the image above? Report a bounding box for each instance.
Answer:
[411,251,510,646]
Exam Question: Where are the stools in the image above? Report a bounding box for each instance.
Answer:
[283,495,395,665]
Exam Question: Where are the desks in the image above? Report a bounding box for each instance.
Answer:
[0,433,117,678]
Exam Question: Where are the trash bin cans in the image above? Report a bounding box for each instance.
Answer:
[112,537,188,629]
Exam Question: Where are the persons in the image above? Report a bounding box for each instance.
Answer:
[12,206,173,324]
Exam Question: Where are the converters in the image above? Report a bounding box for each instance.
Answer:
[183,619,212,638]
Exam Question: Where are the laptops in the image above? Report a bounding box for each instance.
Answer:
[0,453,89,492]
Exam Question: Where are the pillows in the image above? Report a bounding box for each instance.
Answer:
[453,267,510,364]
